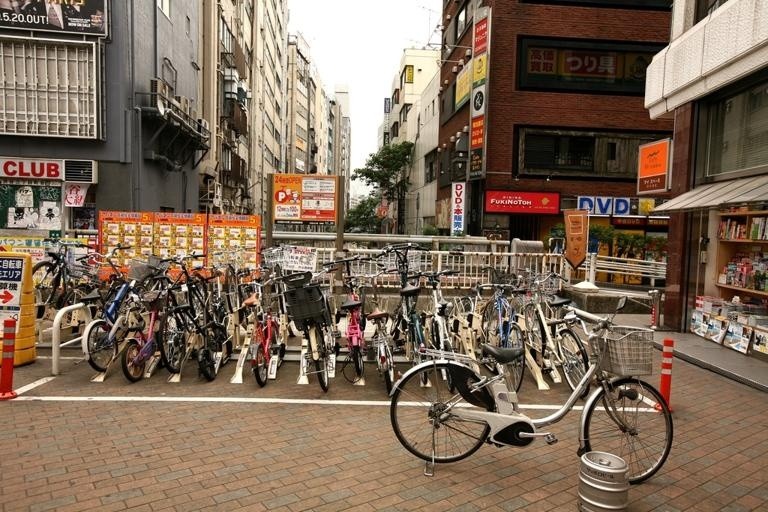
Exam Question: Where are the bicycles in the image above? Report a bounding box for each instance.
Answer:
[30,236,591,398]
[385,297,676,488]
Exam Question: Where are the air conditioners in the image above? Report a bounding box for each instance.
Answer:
[62,158,98,185]
[151,79,211,147]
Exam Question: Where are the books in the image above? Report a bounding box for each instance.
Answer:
[694,294,767,327]
[716,216,767,241]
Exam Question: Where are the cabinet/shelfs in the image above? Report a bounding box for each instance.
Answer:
[713,202,768,355]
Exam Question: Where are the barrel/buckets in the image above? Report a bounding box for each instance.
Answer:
[578,451,627,512]
[0,251,37,366]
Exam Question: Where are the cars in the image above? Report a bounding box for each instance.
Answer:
[345,226,377,234]
